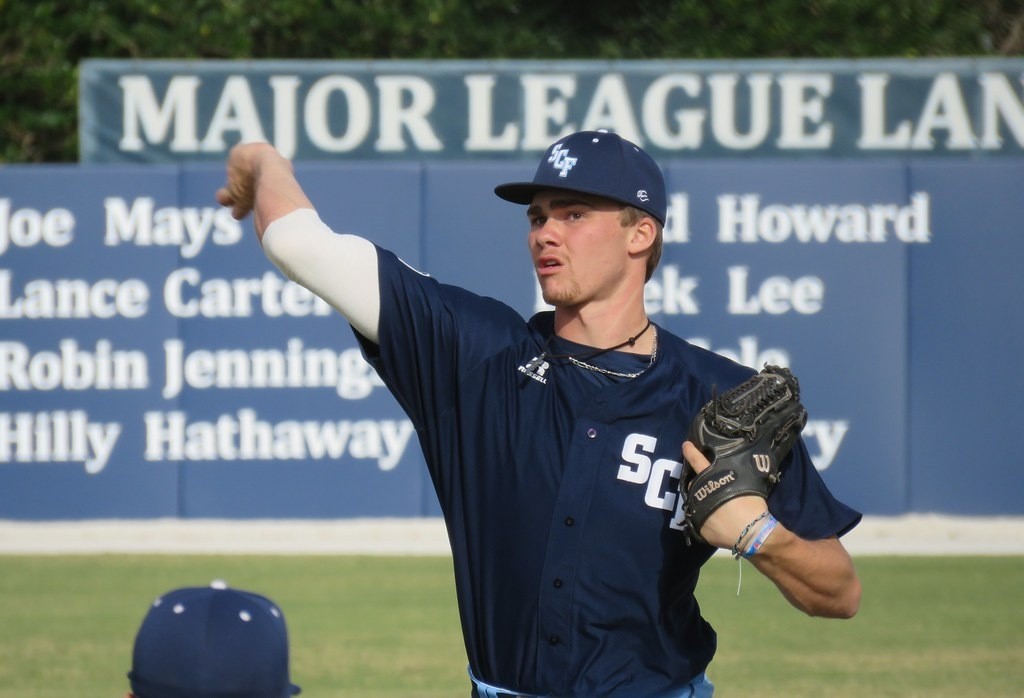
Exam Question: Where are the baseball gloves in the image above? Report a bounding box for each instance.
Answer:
[679,364,809,544]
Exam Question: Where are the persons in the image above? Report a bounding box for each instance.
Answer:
[216,131,862,698]
[123,581,303,698]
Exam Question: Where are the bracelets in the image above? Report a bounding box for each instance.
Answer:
[731,509,777,561]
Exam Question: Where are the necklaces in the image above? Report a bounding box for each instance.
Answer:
[526,317,658,378]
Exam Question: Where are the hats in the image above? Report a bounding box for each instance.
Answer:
[128,581,303,698]
[493,131,667,226]
